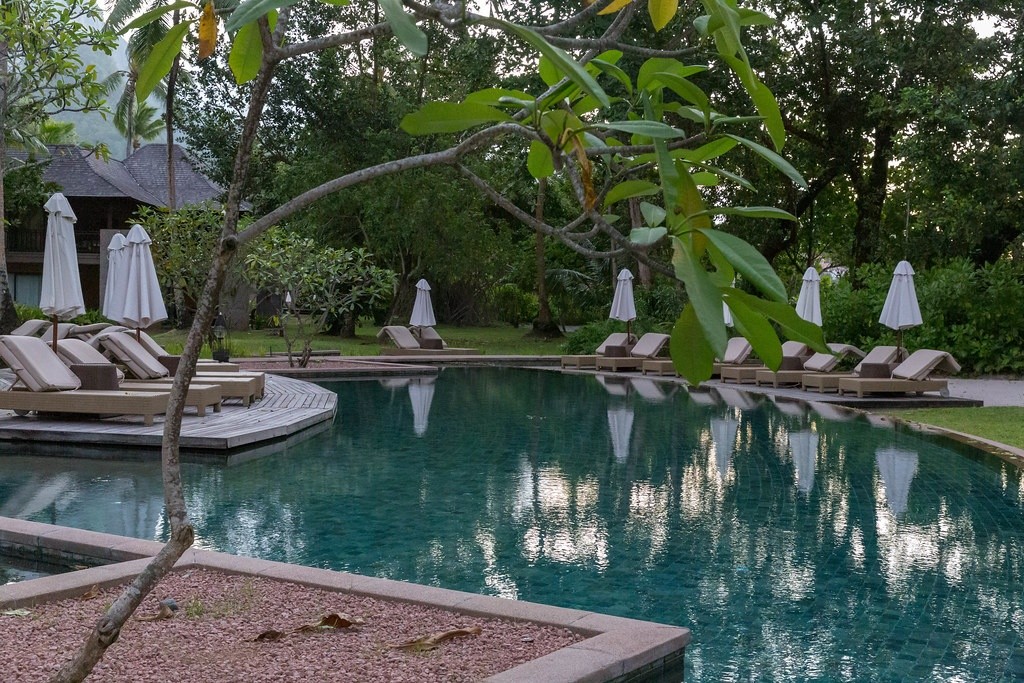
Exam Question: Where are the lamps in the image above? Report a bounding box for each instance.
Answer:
[211,312,232,363]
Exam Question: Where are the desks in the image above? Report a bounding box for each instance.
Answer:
[72,362,121,418]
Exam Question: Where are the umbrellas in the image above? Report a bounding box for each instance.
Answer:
[794,267,824,357]
[407,376,435,437]
[38,193,88,354]
[121,223,169,344]
[409,279,436,348]
[604,383,921,518]
[609,268,637,345]
[878,260,924,363]
[721,277,736,327]
[102,233,127,326]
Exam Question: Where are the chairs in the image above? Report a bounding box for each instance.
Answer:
[838,349,962,400]
[756,343,867,390]
[408,325,448,347]
[48,338,222,415]
[596,333,671,372]
[86,325,130,351]
[802,345,910,393]
[721,341,809,385]
[199,372,265,397]
[10,319,52,337]
[40,324,92,341]
[561,333,639,370]
[378,326,420,349]
[0,336,170,425]
[91,333,257,408]
[713,338,753,374]
[127,330,239,372]
[642,360,674,377]
